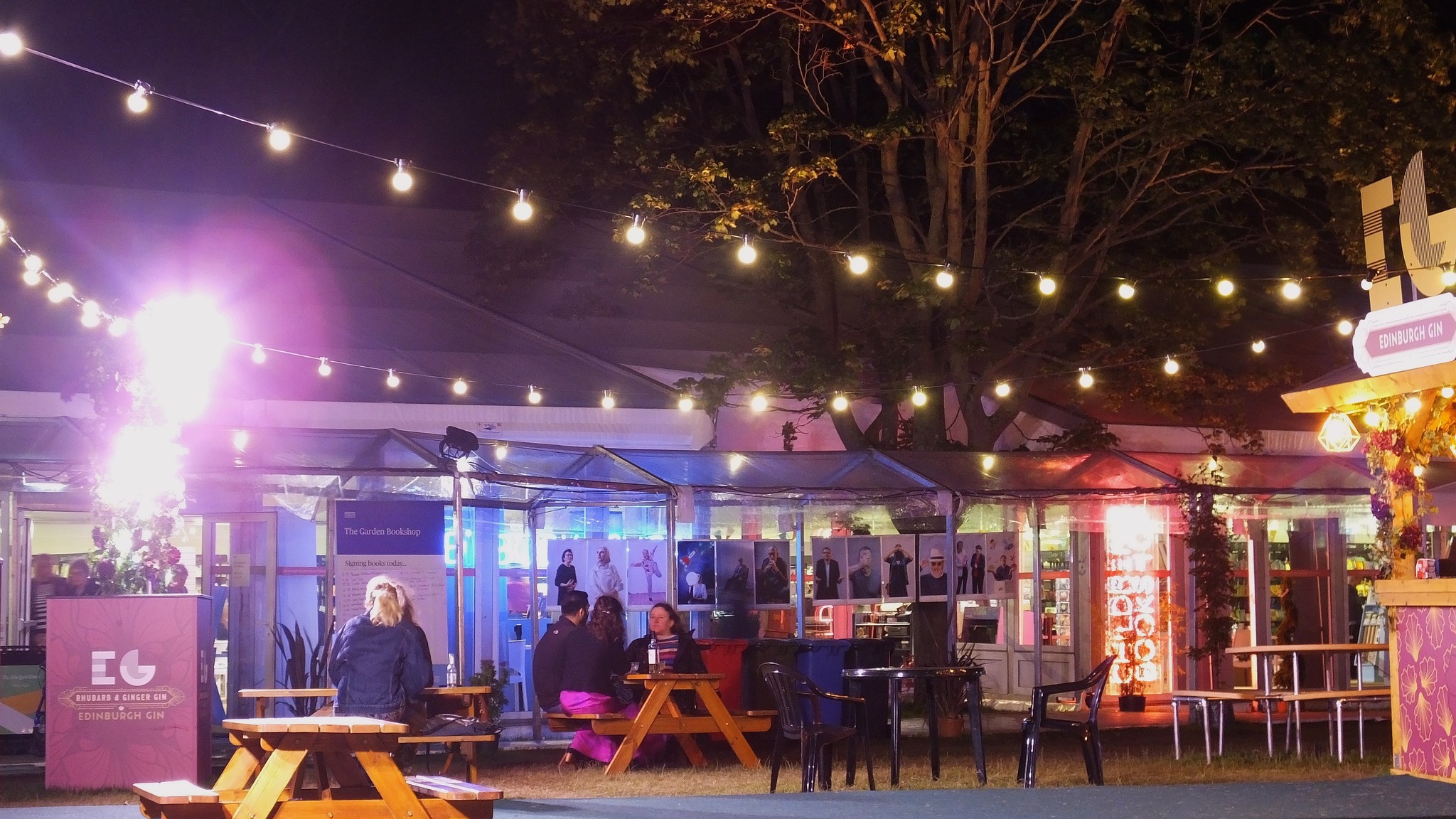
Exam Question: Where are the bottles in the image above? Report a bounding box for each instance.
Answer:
[446,653,456,688]
[648,631,661,674]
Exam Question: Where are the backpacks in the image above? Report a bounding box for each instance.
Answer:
[419,713,505,752]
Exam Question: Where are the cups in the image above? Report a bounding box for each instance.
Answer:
[631,662,640,671]
[660,661,665,671]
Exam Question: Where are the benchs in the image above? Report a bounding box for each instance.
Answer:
[542,710,778,775]
[1171,689,1391,766]
[132,735,504,819]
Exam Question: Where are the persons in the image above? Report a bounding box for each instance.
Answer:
[325,577,435,775]
[498,576,711,774]
[558,540,1016,607]
[1346,584,1366,665]
[31,554,103,654]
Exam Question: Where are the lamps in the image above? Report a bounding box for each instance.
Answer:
[439,426,479,462]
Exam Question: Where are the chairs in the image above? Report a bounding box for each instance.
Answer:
[756,662,866,793]
[1017,653,1118,788]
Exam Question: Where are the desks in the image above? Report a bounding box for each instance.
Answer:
[842,667,987,791]
[239,687,492,784]
[1225,643,1389,761]
[604,674,760,774]
[212,716,431,819]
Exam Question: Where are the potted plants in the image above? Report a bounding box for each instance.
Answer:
[922,642,993,737]
[1175,464,1248,731]
[469,659,510,761]
[1114,581,1152,712]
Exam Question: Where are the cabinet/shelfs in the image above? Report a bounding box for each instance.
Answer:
[852,612,911,651]
